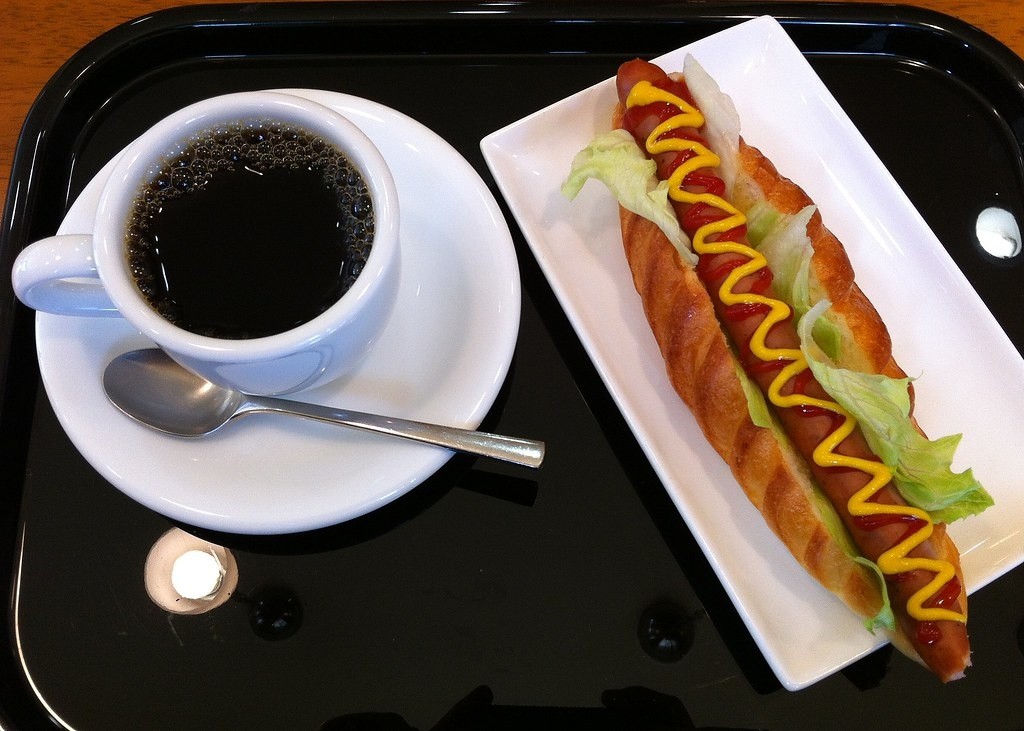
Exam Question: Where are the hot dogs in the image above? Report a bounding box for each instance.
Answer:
[557,51,996,684]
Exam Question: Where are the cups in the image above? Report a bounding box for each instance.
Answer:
[11,90,402,401]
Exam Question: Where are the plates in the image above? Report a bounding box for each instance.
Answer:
[35,87,522,535]
[474,14,1024,693]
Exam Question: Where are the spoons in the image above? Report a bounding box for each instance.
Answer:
[102,348,546,471]
